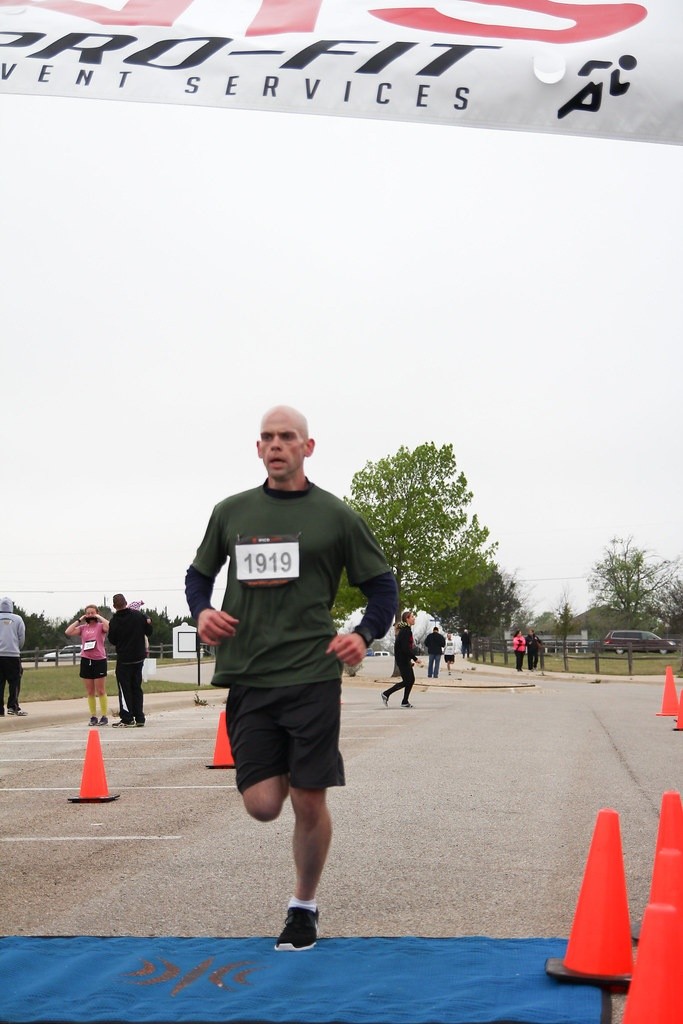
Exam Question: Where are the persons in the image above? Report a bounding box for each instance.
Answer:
[381,612,422,709]
[442,634,457,675]
[0,596,29,716]
[65,604,110,727]
[112,599,145,717]
[512,630,526,672]
[424,626,445,678]
[525,629,543,670]
[107,594,153,728]
[461,629,471,658]
[184,406,398,951]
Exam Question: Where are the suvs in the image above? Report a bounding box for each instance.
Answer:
[43,644,82,661]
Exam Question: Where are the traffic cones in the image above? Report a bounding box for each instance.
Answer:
[621,790,683,1024]
[67,729,120,802]
[205,711,235,768]
[656,666,681,716]
[544,806,633,985]
[672,690,683,730]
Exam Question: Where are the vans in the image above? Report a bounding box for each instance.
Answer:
[374,651,393,656]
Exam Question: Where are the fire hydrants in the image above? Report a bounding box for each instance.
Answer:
[199,648,205,658]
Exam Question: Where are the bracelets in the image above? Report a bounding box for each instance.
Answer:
[76,618,81,623]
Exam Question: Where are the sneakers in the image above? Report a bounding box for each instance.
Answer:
[381,692,388,707]
[88,717,98,726]
[274,907,319,952]
[96,716,109,727]
[112,721,135,728]
[7,707,27,716]
[401,701,416,709]
[0,714,4,719]
[136,721,144,726]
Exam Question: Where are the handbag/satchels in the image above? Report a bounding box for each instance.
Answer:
[535,636,543,649]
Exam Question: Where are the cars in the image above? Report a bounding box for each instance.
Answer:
[365,648,374,656]
[603,630,677,654]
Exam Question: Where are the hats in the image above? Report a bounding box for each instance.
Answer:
[434,627,438,631]
[127,601,144,611]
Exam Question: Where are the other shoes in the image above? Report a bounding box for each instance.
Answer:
[449,671,451,675]
[428,674,432,677]
[434,675,438,678]
